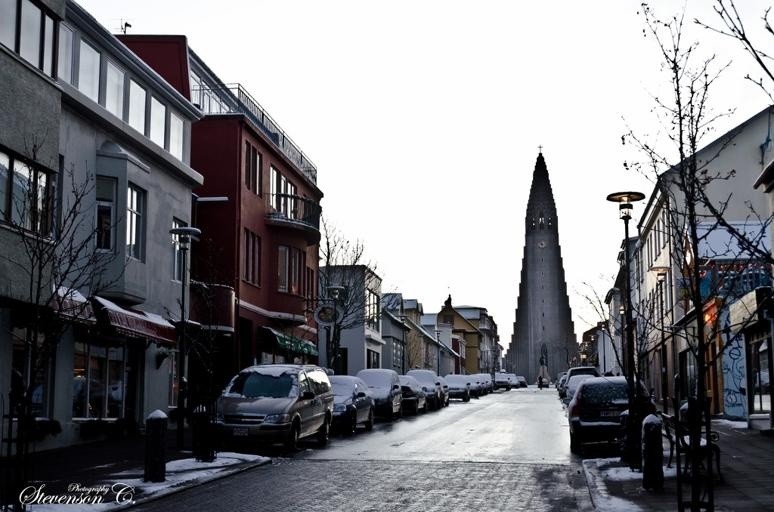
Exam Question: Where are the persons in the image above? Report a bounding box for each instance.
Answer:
[538,376,543,390]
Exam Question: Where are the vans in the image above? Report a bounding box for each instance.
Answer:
[210,364,333,456]
[31,378,111,414]
[359,368,402,422]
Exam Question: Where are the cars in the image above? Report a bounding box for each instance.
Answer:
[327,376,375,436]
[398,375,426,416]
[407,368,528,409]
[555,365,601,408]
[537,378,550,387]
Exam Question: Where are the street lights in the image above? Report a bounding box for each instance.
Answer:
[326,285,346,372]
[434,328,445,377]
[588,334,597,364]
[458,339,465,374]
[618,304,626,374]
[166,225,201,428]
[647,265,673,415]
[394,313,408,375]
[608,190,647,468]
[601,323,608,372]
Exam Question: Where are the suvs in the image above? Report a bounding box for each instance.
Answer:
[562,375,659,457]
